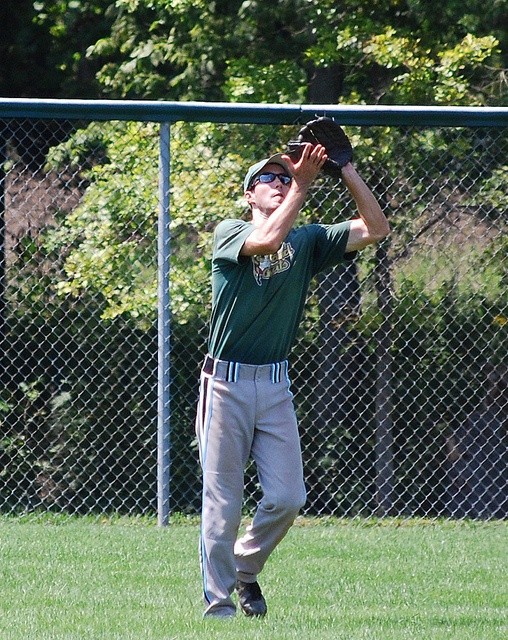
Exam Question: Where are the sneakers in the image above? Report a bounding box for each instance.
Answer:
[237,578,267,616]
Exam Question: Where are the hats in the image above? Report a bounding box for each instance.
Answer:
[244,154,293,192]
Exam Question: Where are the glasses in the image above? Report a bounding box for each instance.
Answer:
[248,172,290,188]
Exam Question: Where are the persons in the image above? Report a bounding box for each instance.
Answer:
[194,115,392,620]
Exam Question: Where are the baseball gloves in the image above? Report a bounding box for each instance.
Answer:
[284,114,352,178]
